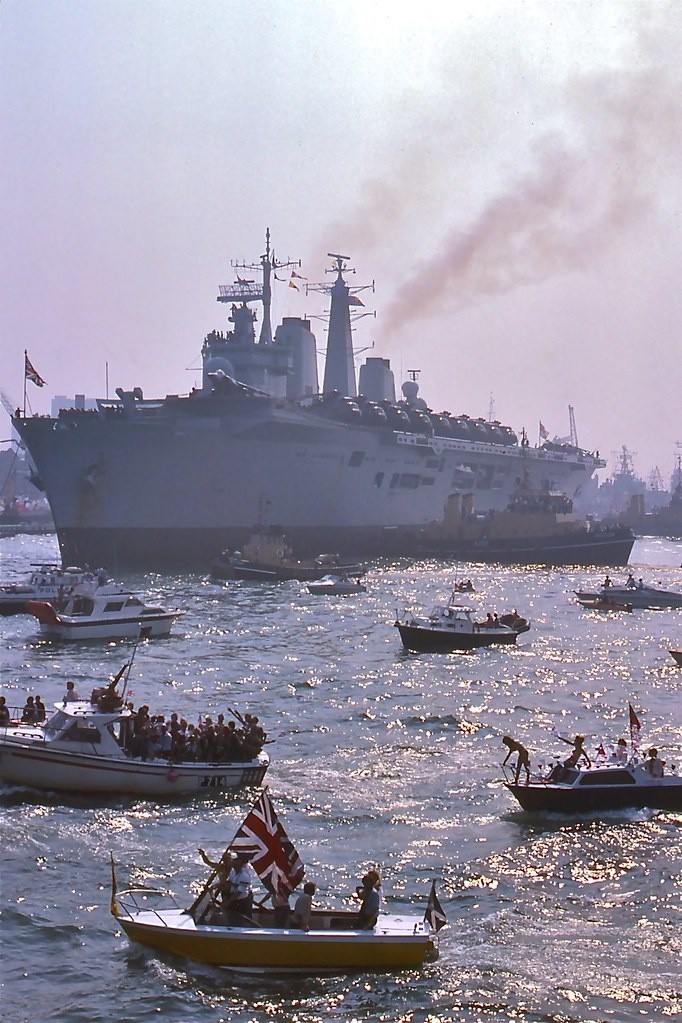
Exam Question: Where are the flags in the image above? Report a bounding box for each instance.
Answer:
[540,424,548,438]
[630,708,641,730]
[425,893,447,934]
[229,794,306,896]
[288,272,301,291]
[25,357,46,387]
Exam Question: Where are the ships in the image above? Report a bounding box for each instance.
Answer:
[10,228,605,569]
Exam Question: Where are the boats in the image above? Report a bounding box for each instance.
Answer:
[0,562,136,616]
[453,578,476,592]
[218,498,371,581]
[634,454,682,537]
[573,584,682,609]
[108,784,446,979]
[390,472,640,569]
[307,570,367,595]
[474,609,533,637]
[668,649,682,667]
[394,562,520,653]
[576,598,634,614]
[0,633,270,801]
[24,579,188,642]
[499,700,681,817]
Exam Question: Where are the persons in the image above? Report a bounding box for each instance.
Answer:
[67,682,77,701]
[545,735,591,780]
[636,748,666,777]
[357,869,381,929]
[58,584,66,605]
[602,576,613,586]
[121,703,275,762]
[463,580,472,589]
[501,736,530,785]
[0,697,9,725]
[485,613,499,627]
[198,849,321,932]
[21,696,45,722]
[16,407,23,418]
[624,574,643,588]
